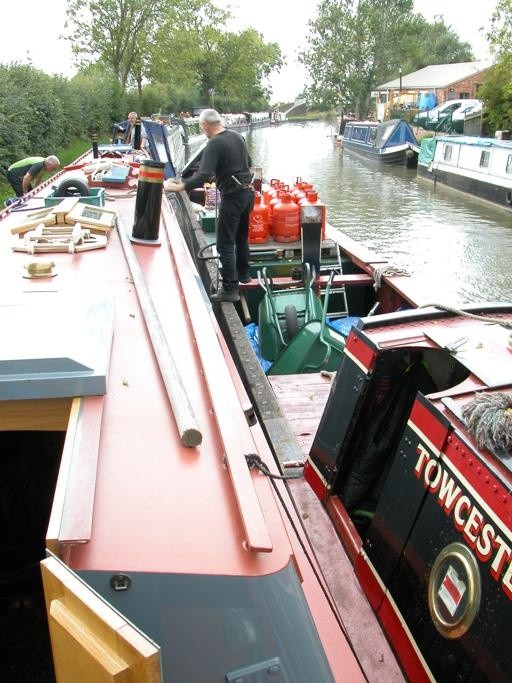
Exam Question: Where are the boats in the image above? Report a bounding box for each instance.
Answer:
[188,157,510,683]
[341,118,420,165]
[414,134,512,202]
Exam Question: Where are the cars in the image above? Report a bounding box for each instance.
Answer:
[413,99,483,132]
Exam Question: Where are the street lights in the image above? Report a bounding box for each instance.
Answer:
[398,64,402,93]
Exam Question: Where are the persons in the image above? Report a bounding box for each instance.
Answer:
[128,110,152,155]
[161,106,257,302]
[6,154,60,199]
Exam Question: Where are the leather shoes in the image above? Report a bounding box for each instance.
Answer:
[210,265,251,302]
